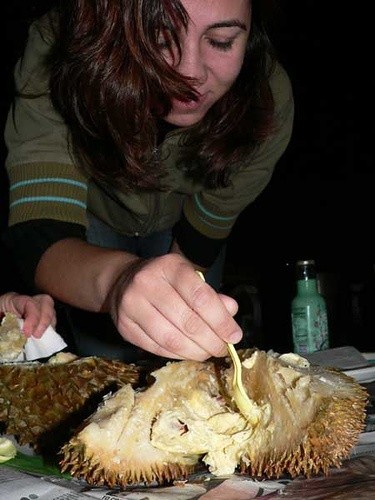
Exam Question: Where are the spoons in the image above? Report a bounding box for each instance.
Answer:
[195,269,258,426]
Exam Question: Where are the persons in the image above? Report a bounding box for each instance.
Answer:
[4,0,295,363]
[0,292,56,339]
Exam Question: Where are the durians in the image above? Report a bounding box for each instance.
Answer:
[0,312,368,487]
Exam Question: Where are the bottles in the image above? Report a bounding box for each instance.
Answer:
[290,260,330,354]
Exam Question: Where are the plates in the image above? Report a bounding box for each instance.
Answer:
[358,414,375,445]
[339,353,375,384]
